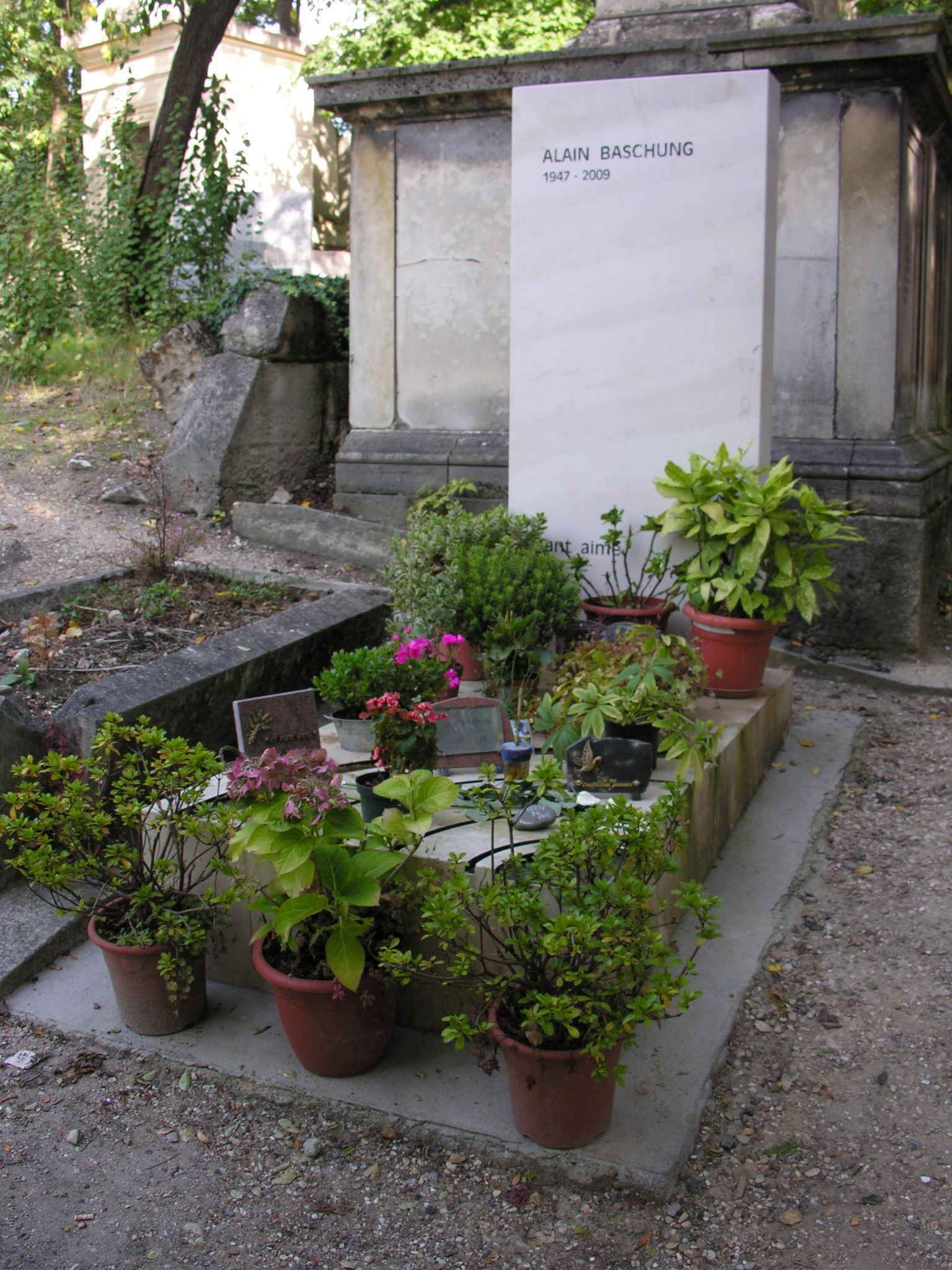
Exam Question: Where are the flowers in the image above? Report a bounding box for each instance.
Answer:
[360,628,465,788]
[229,745,460,1000]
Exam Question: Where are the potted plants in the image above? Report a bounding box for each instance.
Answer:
[0,443,867,1150]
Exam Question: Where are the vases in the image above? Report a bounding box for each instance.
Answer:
[252,929,413,1078]
[354,772,411,824]
